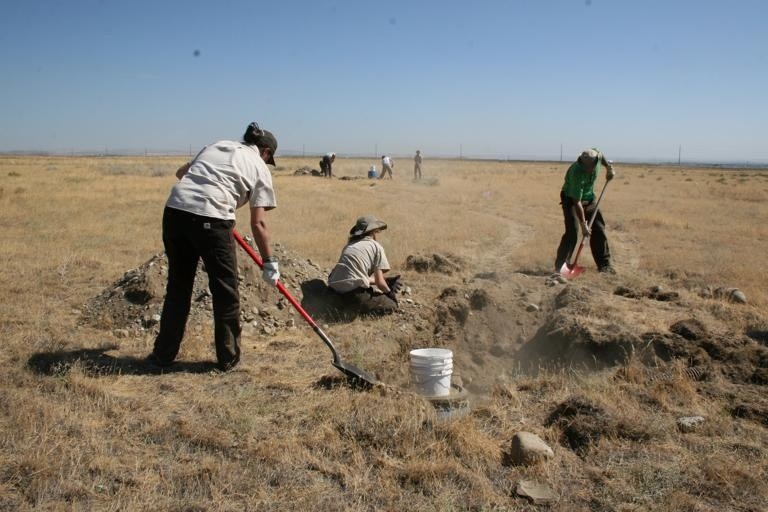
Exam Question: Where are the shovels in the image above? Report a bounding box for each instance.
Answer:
[233,226,385,388]
[560,179,610,281]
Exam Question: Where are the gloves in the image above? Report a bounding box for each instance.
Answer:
[385,274,403,294]
[605,164,615,181]
[580,220,592,238]
[385,290,398,307]
[261,261,280,287]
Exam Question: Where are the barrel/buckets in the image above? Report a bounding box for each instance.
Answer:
[407,348,455,398]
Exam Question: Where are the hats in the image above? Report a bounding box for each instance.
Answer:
[350,215,388,237]
[255,130,278,167]
[577,149,601,172]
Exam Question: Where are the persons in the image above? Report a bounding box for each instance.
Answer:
[555,148,617,274]
[414,151,423,180]
[153,122,280,373]
[320,160,329,174]
[379,155,394,180]
[328,214,403,313]
[323,152,336,178]
